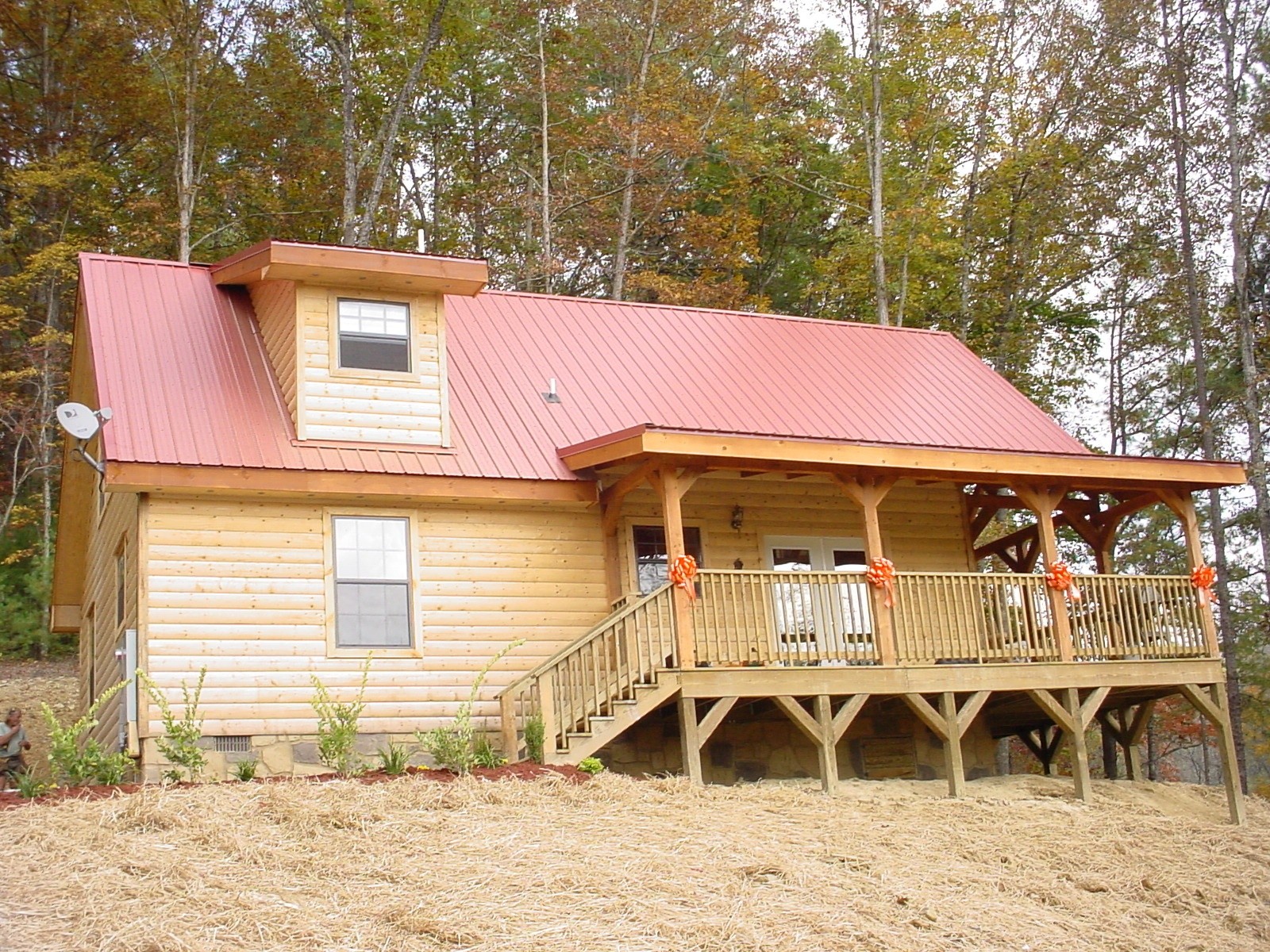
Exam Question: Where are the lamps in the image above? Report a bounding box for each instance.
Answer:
[730,502,744,534]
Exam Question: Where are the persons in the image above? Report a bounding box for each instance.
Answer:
[0,704,34,793]
